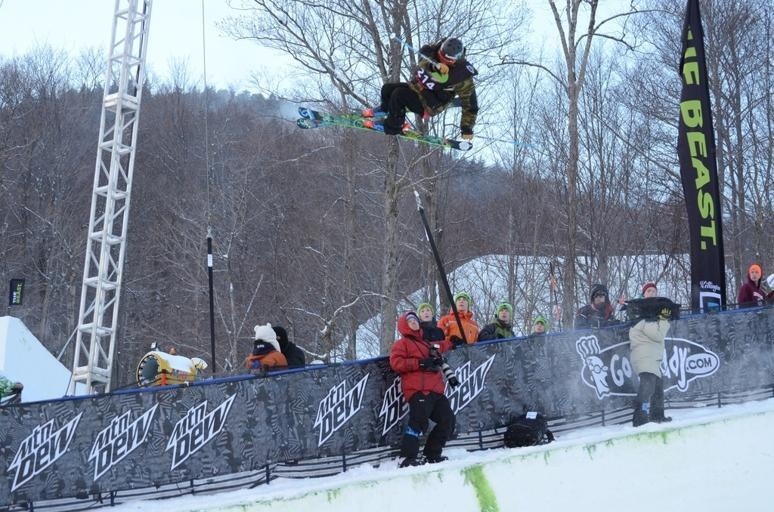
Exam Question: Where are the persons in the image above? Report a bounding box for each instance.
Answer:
[0,382,24,406]
[531,316,550,335]
[738,264,774,308]
[477,303,516,343]
[374,38,480,140]
[243,321,287,370]
[169,347,179,355]
[390,311,457,468]
[438,292,479,344]
[573,281,672,427]
[273,327,305,369]
[416,303,449,352]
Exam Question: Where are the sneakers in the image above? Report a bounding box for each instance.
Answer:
[364,118,409,133]
[362,105,390,117]
[398,450,449,468]
[632,408,672,427]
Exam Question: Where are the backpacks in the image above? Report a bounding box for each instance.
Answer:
[503,404,556,448]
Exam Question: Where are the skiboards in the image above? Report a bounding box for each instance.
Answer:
[295,106,473,151]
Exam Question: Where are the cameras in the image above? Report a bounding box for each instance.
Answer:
[433,357,461,391]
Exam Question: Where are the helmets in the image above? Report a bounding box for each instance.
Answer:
[437,36,465,66]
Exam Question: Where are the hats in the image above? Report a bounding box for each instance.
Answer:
[749,264,762,276]
[590,284,609,300]
[271,326,288,348]
[416,302,435,320]
[766,274,774,290]
[454,292,472,307]
[533,316,549,333]
[641,281,657,296]
[495,303,514,319]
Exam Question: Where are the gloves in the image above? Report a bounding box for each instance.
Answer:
[419,347,444,373]
[461,127,475,139]
[658,308,672,320]
[436,63,450,74]
